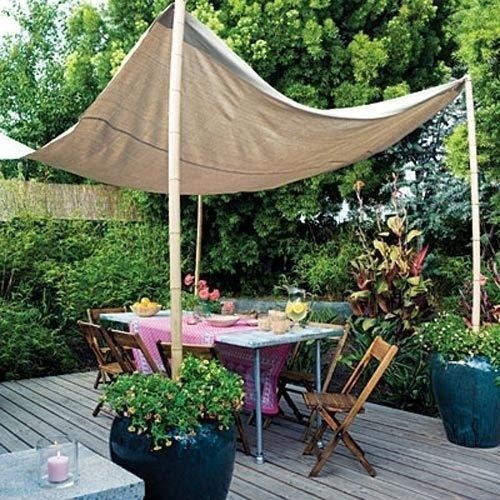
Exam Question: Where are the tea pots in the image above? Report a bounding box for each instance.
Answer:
[283,283,314,332]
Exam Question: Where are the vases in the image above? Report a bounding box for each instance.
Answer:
[431,349,500,447]
[109,413,237,500]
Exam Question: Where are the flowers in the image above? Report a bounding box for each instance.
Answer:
[413,310,500,386]
[99,350,247,451]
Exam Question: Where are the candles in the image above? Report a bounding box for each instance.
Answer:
[47,451,69,483]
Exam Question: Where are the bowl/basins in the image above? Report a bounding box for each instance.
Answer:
[130,305,162,317]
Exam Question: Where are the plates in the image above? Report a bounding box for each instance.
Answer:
[205,314,258,326]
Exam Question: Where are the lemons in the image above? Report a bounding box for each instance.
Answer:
[293,304,306,314]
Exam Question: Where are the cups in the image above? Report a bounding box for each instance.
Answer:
[36,436,78,489]
[259,310,290,334]
